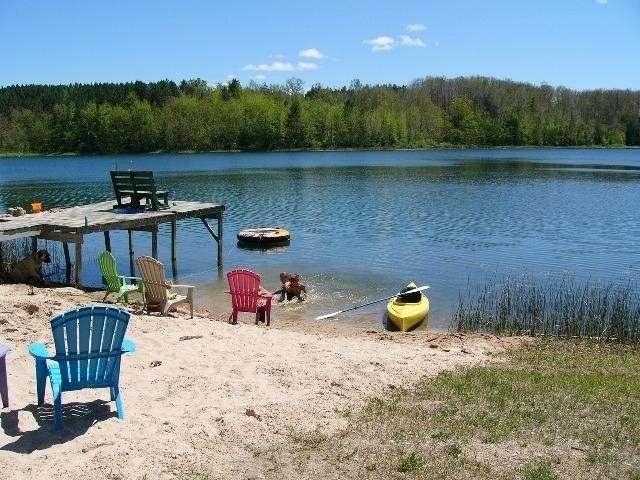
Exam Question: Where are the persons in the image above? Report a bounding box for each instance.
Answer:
[273,272,308,302]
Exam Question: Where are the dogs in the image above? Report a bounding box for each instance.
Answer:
[7,250,52,284]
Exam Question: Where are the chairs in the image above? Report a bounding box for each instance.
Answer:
[32,305,136,432]
[98,252,193,320]
[224,270,273,326]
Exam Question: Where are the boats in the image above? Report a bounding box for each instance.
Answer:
[237,227,291,244]
[386,281,429,332]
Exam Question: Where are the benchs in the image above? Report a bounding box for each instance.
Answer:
[110,170,170,211]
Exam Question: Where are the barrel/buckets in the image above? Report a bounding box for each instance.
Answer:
[31,202,42,213]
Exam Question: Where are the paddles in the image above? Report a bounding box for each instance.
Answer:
[314,286,430,321]
